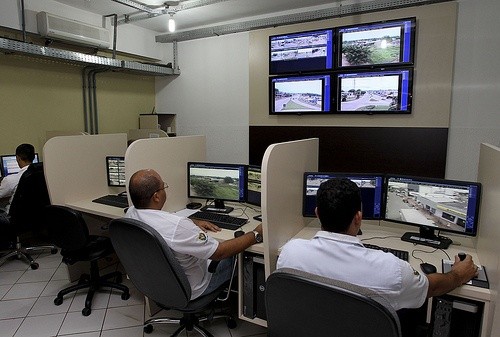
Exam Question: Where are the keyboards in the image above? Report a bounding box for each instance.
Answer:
[93,195,129,208]
[186,211,248,230]
[363,243,409,263]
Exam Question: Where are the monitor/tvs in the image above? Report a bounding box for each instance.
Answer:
[384,175,482,249]
[335,16,416,70]
[336,66,414,113]
[268,27,338,74]
[269,72,335,115]
[245,165,261,208]
[106,155,126,186]
[0,153,39,178]
[302,173,386,235]
[185,161,247,213]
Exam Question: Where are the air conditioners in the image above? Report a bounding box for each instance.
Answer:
[37,11,110,49]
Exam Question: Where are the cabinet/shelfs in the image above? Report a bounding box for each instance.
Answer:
[139,113,176,137]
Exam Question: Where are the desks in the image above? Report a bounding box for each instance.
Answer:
[42,133,500,337]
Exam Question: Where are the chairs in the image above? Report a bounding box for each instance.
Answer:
[0,162,56,270]
[264,267,402,337]
[108,217,237,337]
[44,204,130,316]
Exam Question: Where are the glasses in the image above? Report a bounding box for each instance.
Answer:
[151,182,168,197]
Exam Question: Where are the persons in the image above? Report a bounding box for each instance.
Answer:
[0,143,38,224]
[125,169,262,311]
[276,179,479,313]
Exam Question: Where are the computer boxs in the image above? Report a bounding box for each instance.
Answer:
[425,295,485,337]
[242,253,266,322]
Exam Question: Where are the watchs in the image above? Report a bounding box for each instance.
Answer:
[251,230,262,243]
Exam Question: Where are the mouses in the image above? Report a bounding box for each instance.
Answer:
[420,262,437,275]
[234,231,244,239]
